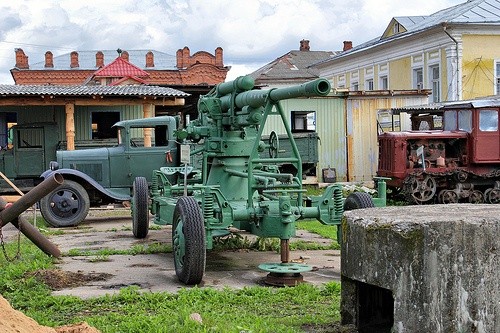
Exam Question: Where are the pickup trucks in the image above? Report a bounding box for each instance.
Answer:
[38,112,322,228]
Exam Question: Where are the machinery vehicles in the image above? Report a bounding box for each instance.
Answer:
[129,77,393,286]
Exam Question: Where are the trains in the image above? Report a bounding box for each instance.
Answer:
[374,94,500,204]
